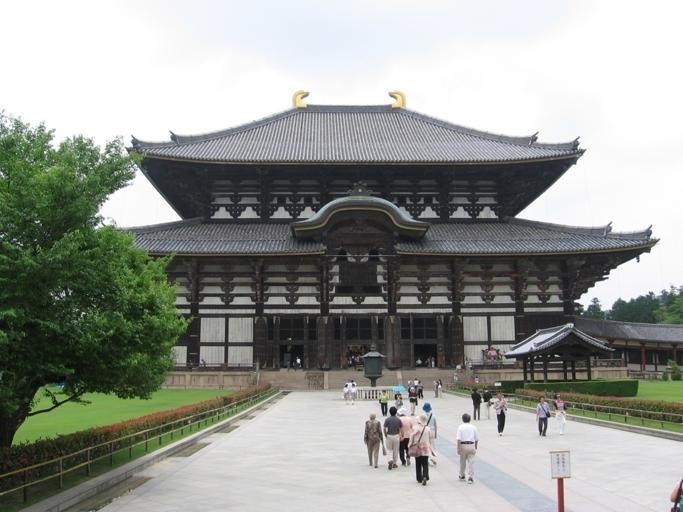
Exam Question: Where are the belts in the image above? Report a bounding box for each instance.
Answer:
[461,442,475,444]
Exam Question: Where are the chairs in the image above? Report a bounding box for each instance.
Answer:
[482,348,504,368]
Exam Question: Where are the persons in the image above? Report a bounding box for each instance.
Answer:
[494,391,507,438]
[341,374,494,471]
[670,477,683,512]
[553,392,567,436]
[455,413,479,485]
[407,414,435,486]
[282,338,506,370]
[535,395,550,439]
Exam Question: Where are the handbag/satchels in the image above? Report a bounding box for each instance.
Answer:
[408,445,421,458]
[382,445,387,456]
[545,410,552,418]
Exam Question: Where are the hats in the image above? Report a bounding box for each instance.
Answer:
[397,408,408,416]
[416,415,428,425]
[422,401,433,411]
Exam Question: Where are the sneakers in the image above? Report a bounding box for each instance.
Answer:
[416,477,427,486]
[388,455,410,470]
[458,475,475,484]
